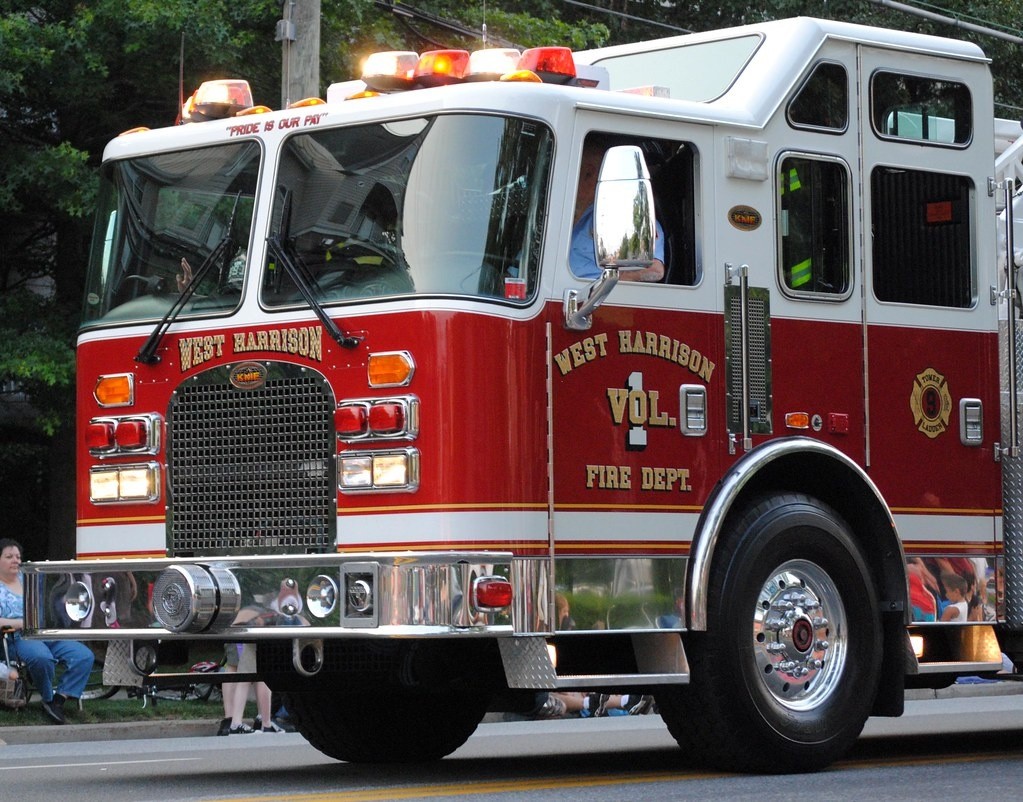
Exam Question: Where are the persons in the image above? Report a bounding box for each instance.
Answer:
[506,140,665,292]
[905,555,1008,624]
[0,537,94,724]
[175,257,201,295]
[533,687,660,720]
[215,641,286,736]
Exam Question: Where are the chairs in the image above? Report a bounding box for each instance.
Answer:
[0,626,58,711]
[278,182,411,292]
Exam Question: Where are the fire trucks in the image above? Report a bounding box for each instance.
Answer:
[9,12,1023,774]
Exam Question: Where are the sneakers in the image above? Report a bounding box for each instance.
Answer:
[262,723,285,734]
[228,725,263,737]
[624,694,649,715]
[587,692,610,717]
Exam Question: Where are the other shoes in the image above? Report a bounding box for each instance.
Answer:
[44,703,65,723]
[253,716,262,731]
[540,697,567,717]
[217,718,232,736]
